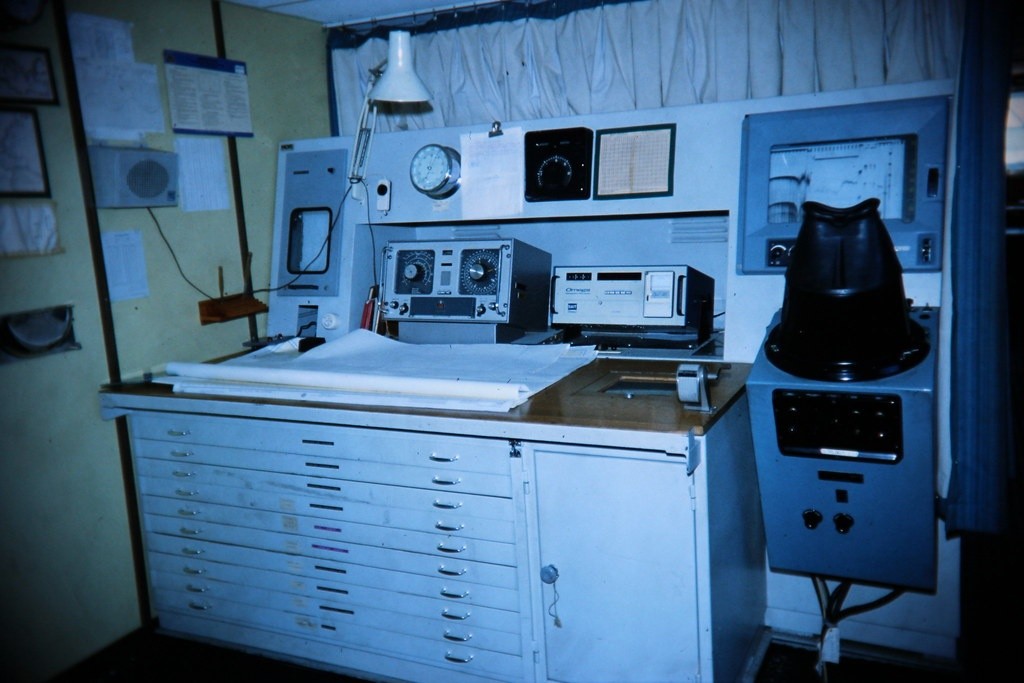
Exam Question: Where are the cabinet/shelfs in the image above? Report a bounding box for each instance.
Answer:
[99,348,769,683]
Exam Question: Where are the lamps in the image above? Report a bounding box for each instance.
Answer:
[347,30,431,206]
